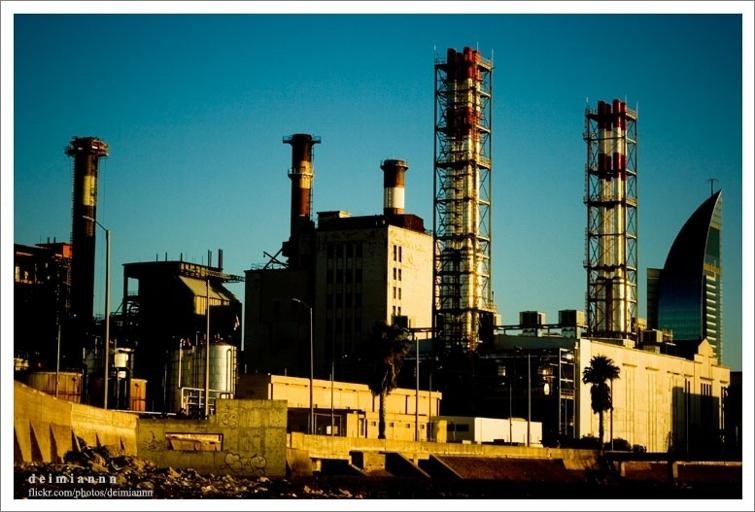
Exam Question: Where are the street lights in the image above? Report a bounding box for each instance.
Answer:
[291,297,317,433]
[82,215,112,412]
[400,325,423,443]
[514,344,533,447]
